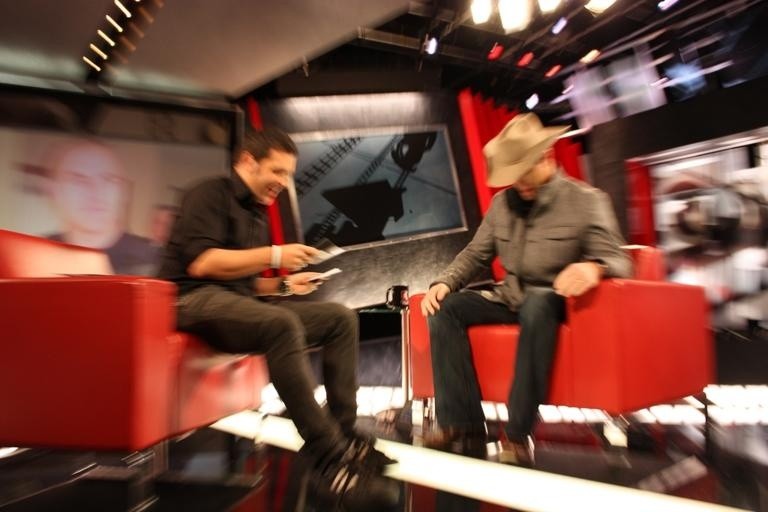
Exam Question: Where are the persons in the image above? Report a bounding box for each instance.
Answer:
[418,112,631,464]
[146,181,184,242]
[157,131,397,500]
[656,169,768,330]
[33,136,163,267]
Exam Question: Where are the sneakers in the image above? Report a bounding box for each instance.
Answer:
[495,436,536,466]
[412,418,490,454]
[309,430,401,508]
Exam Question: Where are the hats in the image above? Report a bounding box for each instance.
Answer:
[479,110,572,190]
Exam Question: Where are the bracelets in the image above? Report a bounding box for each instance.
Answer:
[277,277,290,297]
[270,243,284,270]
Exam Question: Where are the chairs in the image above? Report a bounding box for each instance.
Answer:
[387,242,717,448]
[0,230,272,466]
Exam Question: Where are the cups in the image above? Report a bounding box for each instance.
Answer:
[385,283,411,310]
